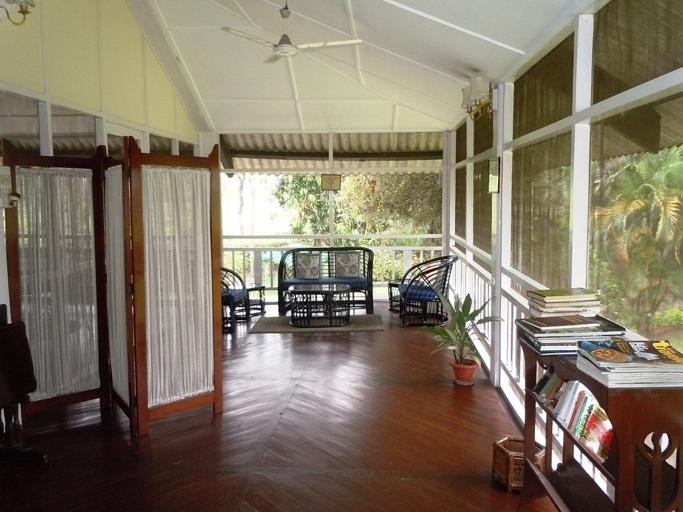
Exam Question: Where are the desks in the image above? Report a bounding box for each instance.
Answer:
[516,333,683,512]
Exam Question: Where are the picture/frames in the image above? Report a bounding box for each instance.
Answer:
[487,157,500,193]
[321,174,341,191]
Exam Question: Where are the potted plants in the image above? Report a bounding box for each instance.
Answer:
[422,292,504,385]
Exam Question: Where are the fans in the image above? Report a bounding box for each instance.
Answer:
[221,1,362,63]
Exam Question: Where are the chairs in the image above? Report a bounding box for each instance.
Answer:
[277,246,374,316]
[221,268,249,334]
[398,255,458,328]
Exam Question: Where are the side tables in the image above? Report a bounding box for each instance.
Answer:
[230,283,267,316]
[388,279,424,312]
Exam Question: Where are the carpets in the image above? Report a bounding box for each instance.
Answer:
[249,314,384,331]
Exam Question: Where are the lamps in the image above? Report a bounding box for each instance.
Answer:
[459,77,495,121]
[1,1,35,25]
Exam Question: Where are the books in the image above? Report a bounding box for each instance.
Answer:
[577,342,683,390]
[527,289,601,317]
[515,315,625,355]
[533,369,612,464]
